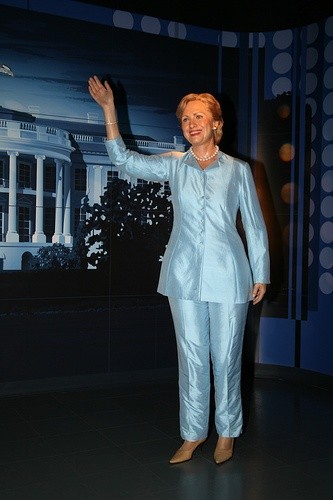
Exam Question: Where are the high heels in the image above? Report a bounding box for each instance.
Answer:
[214,436,234,464]
[170,436,207,463]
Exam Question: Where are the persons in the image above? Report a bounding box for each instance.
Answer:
[88,75,271,464]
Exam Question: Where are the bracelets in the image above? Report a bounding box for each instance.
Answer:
[104,121,117,126]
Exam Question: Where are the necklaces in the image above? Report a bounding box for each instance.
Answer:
[189,145,219,161]
